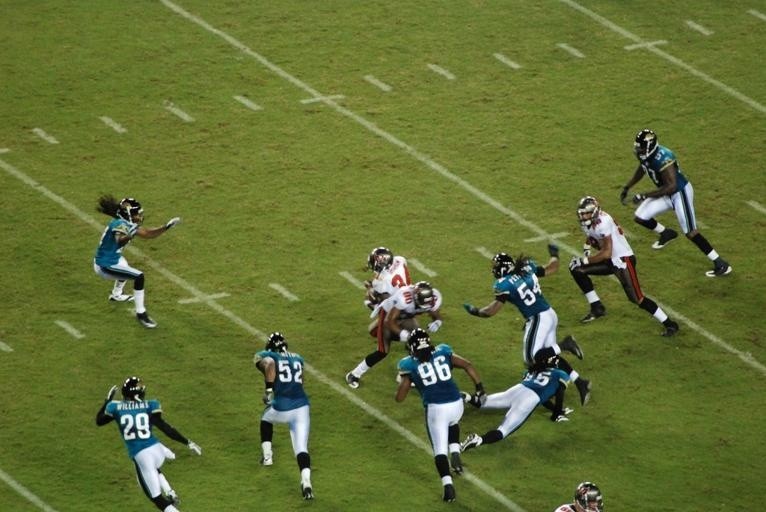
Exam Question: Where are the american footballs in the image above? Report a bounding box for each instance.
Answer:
[589,237,597,247]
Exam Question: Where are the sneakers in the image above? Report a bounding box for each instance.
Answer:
[301,480,313,499]
[651,229,677,249]
[442,391,481,503]
[705,264,732,277]
[261,457,273,465]
[582,306,605,323]
[166,490,177,506]
[137,311,156,328]
[661,322,678,336]
[565,335,590,405]
[346,372,359,388]
[109,294,134,302]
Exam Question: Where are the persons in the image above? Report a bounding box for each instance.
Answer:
[345,279,444,390]
[621,124,733,276]
[395,327,487,502]
[367,243,414,345]
[90,190,179,332]
[456,348,572,459]
[95,374,204,511]
[462,240,593,407]
[253,331,315,505]
[551,481,608,512]
[567,193,681,336]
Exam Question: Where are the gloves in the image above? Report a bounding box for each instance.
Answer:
[548,243,558,256]
[165,217,179,229]
[129,224,137,237]
[399,319,442,342]
[264,389,273,405]
[633,193,645,203]
[569,244,592,271]
[107,385,118,400]
[475,391,486,404]
[555,406,574,421]
[463,304,479,315]
[188,439,202,455]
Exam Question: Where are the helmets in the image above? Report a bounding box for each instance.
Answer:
[633,130,659,160]
[123,377,144,398]
[492,253,513,278]
[408,328,432,354]
[413,281,434,309]
[367,247,392,273]
[546,355,560,366]
[574,481,603,512]
[576,196,599,226]
[266,332,288,353]
[116,197,144,225]
